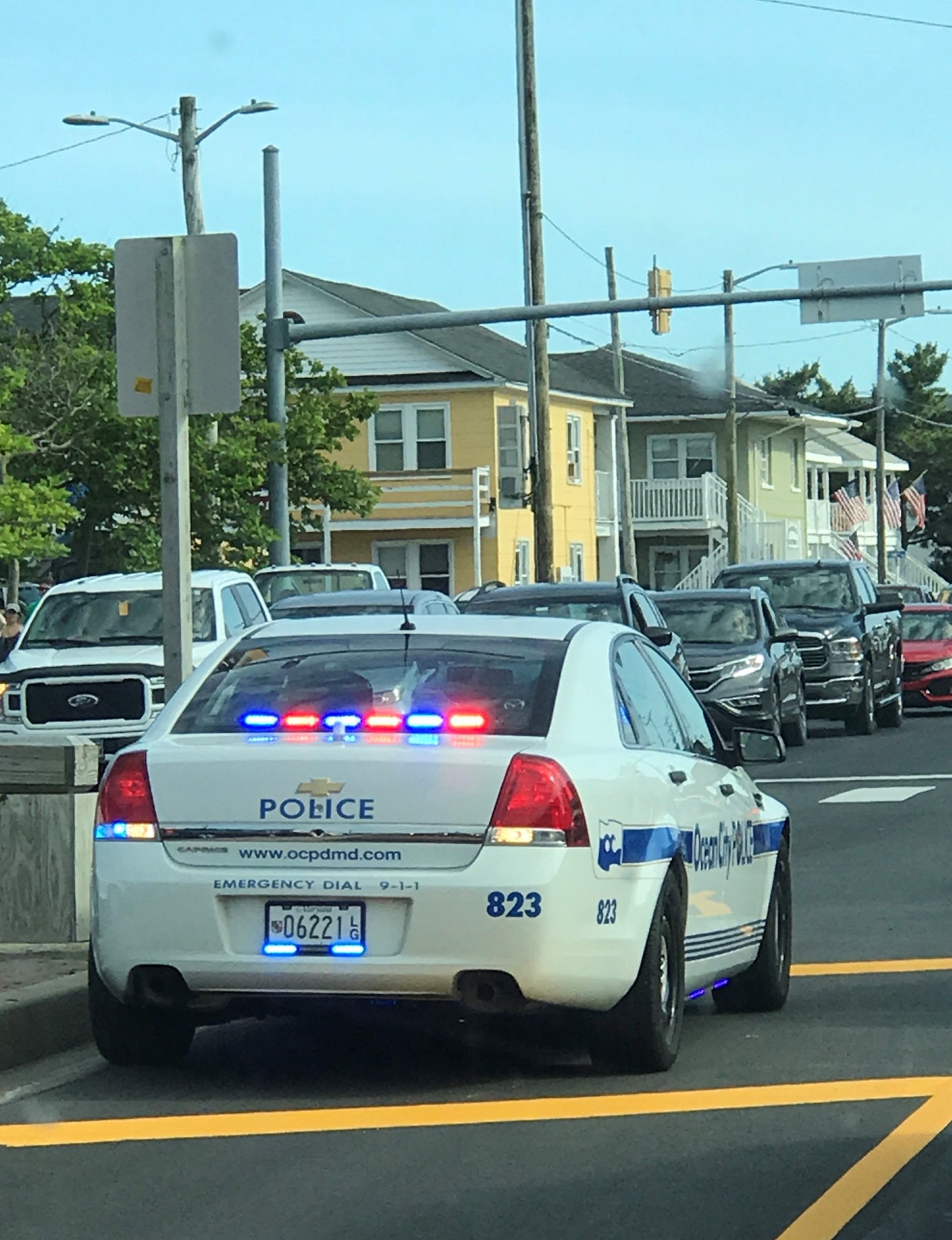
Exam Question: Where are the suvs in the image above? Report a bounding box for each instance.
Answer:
[250,561,393,612]
[710,558,907,737]
[645,584,809,749]
[462,573,691,689]
[0,567,275,777]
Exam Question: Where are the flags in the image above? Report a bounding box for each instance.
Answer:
[882,481,902,530]
[830,502,851,532]
[831,481,870,524]
[904,475,926,534]
[836,531,863,562]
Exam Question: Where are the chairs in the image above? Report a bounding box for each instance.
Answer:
[276,674,374,719]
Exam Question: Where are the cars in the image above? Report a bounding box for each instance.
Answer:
[872,584,952,711]
[263,587,461,619]
[83,611,798,1072]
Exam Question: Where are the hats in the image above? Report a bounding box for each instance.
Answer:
[5,603,21,613]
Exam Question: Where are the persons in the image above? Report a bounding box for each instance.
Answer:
[0,602,25,663]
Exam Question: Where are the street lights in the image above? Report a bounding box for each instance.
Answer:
[715,260,805,566]
[872,305,951,583]
[61,94,279,525]
